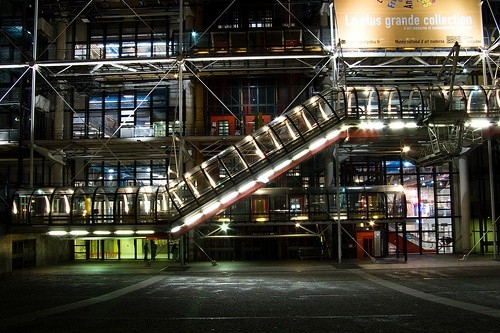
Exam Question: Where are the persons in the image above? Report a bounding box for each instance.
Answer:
[171,243,179,261]
[151,241,157,261]
[144,242,149,262]
